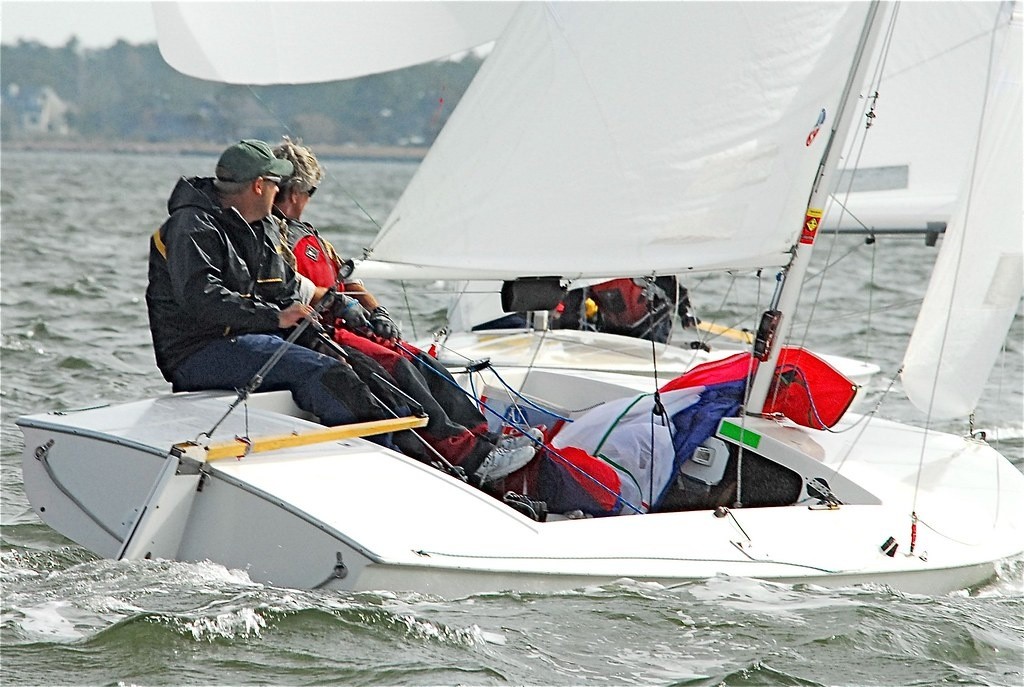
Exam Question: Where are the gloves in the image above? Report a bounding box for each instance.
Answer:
[333,293,370,327]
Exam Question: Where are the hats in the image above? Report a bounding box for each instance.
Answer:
[217,138,294,182]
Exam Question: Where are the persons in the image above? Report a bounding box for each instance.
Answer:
[272,135,545,486]
[145,138,464,481]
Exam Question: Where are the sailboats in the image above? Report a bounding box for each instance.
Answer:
[14,3,1024,603]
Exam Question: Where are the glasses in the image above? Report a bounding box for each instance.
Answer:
[307,186,317,197]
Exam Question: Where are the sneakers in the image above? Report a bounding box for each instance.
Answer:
[475,446,535,484]
[497,427,544,452]
